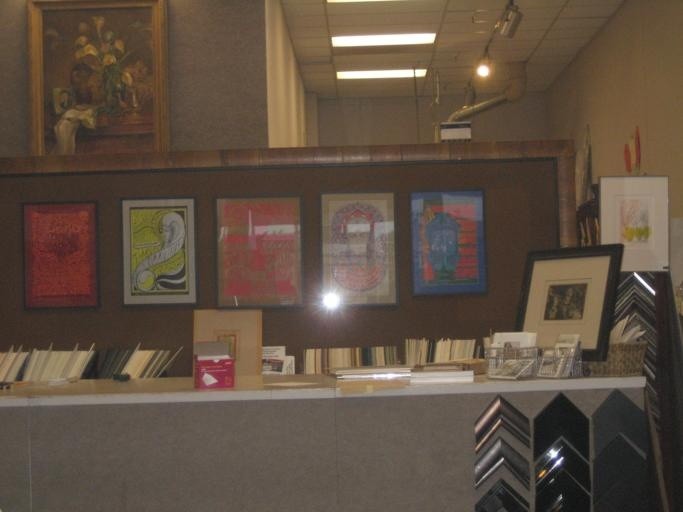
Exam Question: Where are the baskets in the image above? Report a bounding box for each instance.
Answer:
[586,340,647,377]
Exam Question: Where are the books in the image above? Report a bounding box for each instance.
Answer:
[304,346,411,382]
[0,342,185,383]
[262,346,295,376]
[405,331,580,383]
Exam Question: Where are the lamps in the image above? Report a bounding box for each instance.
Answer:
[462,0,524,111]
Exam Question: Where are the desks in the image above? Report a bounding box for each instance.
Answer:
[1,374,650,512]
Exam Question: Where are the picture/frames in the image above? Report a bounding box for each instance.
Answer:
[317,190,400,310]
[24,0,170,157]
[598,174,671,275]
[119,192,200,308]
[20,199,101,312]
[213,190,306,312]
[409,188,489,298]
[514,243,624,362]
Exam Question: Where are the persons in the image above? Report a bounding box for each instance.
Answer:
[557,287,578,319]
